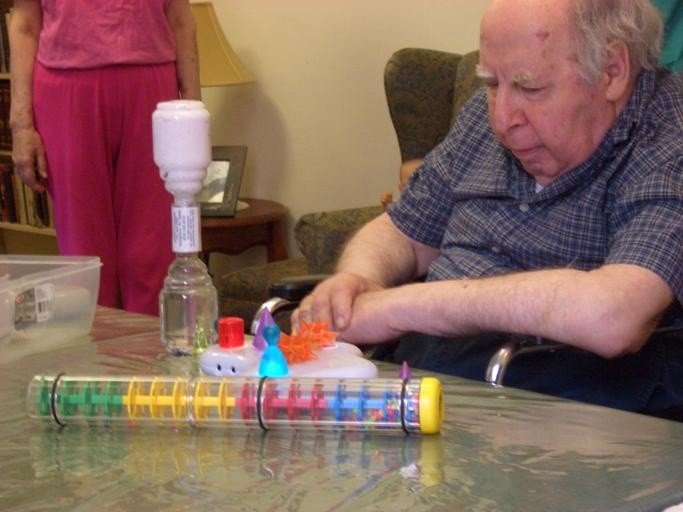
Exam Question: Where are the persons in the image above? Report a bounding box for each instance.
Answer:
[5,1,206,320]
[280,1,681,380]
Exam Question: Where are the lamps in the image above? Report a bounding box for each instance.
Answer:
[186,0,257,90]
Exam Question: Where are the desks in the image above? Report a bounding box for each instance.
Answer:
[198,199,287,276]
[0,307,682,512]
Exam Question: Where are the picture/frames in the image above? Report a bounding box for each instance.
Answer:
[197,146,246,220]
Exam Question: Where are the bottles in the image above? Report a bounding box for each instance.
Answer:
[149,95,222,356]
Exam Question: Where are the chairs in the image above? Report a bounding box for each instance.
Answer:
[209,44,480,338]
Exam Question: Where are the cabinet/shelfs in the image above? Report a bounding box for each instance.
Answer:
[0,0,62,257]
